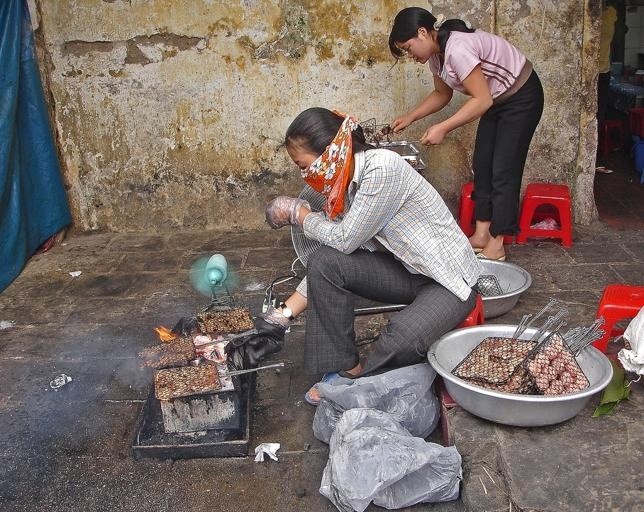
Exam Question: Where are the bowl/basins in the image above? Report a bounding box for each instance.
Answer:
[475,255,533,319]
[424,323,615,429]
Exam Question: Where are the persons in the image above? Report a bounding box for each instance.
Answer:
[218,107,485,407]
[390,7,546,263]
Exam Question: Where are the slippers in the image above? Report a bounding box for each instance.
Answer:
[464,240,506,265]
[306,366,364,407]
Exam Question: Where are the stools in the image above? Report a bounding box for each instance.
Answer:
[591,281,643,353]
[458,184,517,244]
[436,294,484,408]
[516,182,573,248]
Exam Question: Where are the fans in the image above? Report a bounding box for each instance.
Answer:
[186,252,243,317]
